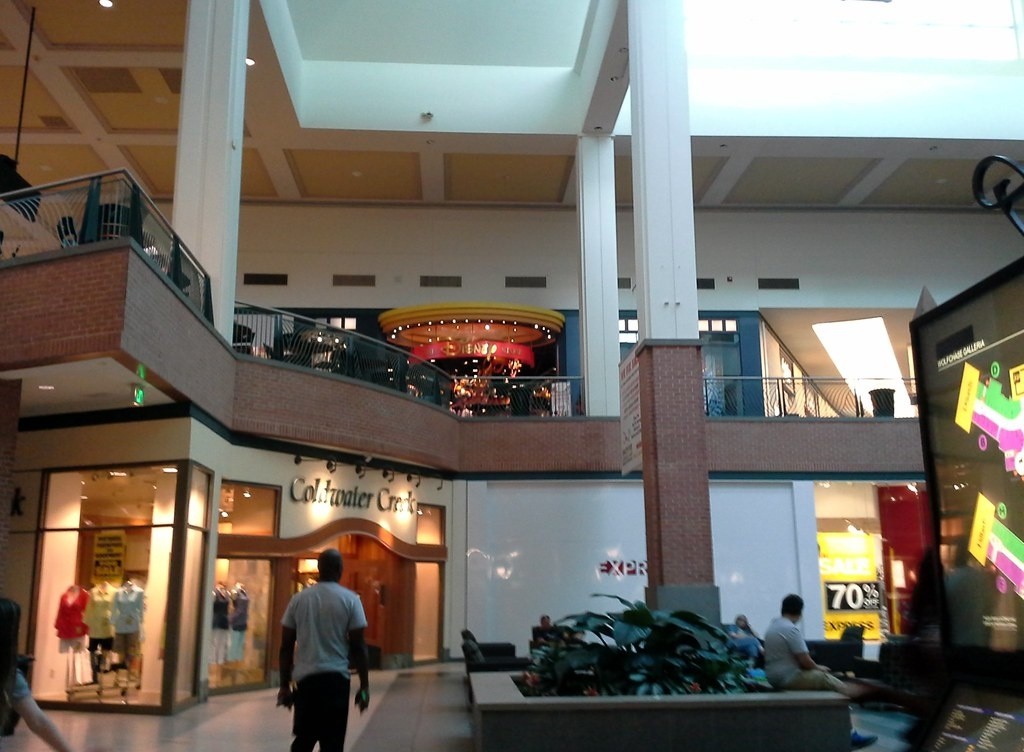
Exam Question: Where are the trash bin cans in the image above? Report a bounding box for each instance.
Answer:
[96,202,133,240]
[869,387,897,418]
[509,384,530,417]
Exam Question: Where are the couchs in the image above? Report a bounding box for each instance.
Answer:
[805,626,864,678]
[461,628,515,658]
[462,640,530,703]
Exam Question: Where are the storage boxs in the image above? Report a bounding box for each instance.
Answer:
[869,387,896,418]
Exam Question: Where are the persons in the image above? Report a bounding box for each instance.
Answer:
[110,579,148,701]
[82,580,121,696]
[908,537,1003,748]
[209,581,232,684]
[275,547,371,751]
[56,582,95,696]
[0,596,72,752]
[763,593,880,752]
[227,581,250,682]
[535,614,558,641]
[726,614,767,671]
[894,543,952,750]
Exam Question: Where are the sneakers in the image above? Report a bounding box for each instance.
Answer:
[852,730,878,749]
